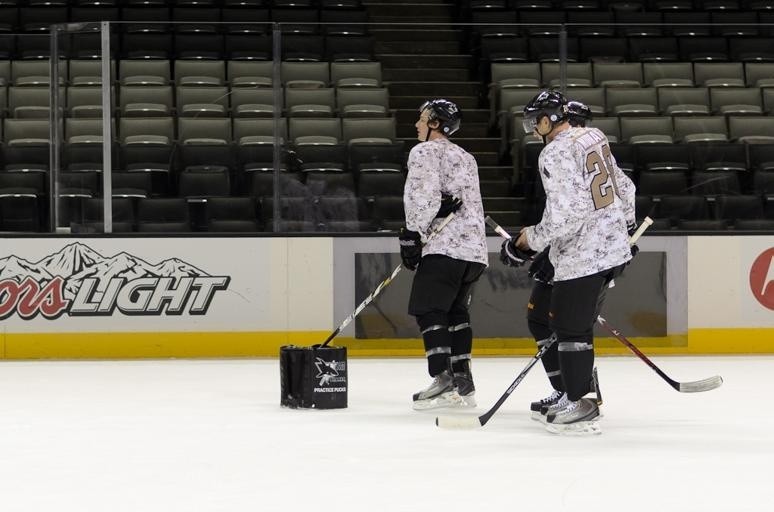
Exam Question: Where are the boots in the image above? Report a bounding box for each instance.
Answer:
[432,371,476,399]
[412,368,459,402]
[530,389,600,425]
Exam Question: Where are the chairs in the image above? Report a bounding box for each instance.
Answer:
[586,115,773,229]
[500,85,545,219]
[2,3,375,62]
[482,0,774,113]
[0,61,398,234]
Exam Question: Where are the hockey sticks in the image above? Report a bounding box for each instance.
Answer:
[485,217,724,392]
[436,217,655,428]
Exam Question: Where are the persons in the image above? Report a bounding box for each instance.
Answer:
[505,91,639,424]
[398,97,488,402]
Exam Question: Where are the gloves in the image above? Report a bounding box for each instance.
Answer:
[499,233,538,268]
[399,227,423,272]
[528,244,556,283]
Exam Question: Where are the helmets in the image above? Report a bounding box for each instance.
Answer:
[563,101,593,122]
[522,89,571,134]
[418,99,461,137]
